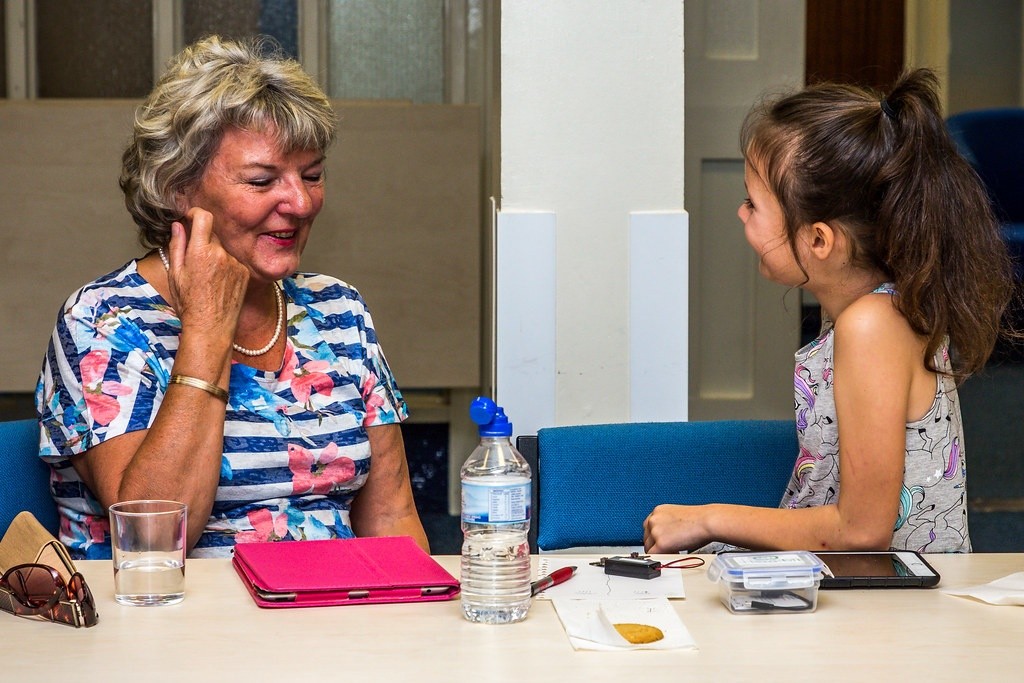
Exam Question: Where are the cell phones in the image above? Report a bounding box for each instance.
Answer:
[809,549,940,590]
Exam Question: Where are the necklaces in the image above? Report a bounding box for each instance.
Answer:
[158,248,283,356]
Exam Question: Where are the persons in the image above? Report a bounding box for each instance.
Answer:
[643,68,1013,554]
[36,34,431,561]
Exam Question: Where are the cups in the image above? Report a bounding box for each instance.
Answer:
[108,499,188,607]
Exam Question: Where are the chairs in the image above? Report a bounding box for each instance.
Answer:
[946,108,1024,280]
[515,418,804,556]
[0,420,59,541]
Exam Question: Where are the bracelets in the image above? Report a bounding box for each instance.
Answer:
[168,375,229,404]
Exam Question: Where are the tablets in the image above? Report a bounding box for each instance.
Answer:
[230,550,451,599]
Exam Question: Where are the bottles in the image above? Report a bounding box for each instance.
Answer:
[460,397,533,624]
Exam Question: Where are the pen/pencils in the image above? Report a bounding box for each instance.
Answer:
[531,566,578,596]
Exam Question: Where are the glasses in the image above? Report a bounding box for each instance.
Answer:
[0,563,99,628]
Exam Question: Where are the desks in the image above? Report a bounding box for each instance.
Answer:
[0,553,1024,683]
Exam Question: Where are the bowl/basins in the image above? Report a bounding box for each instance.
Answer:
[707,550,835,614]
